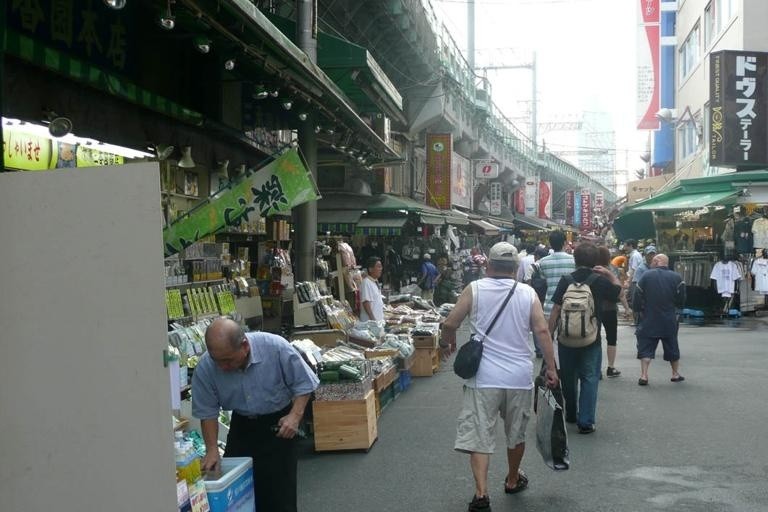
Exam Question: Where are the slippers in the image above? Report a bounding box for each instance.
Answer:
[671,376,684,381]
[639,377,648,385]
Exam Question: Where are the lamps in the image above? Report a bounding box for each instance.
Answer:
[157,2,175,29]
[108,0,127,9]
[147,121,250,180]
[224,39,237,70]
[634,107,703,180]
[195,13,212,52]
[44,106,72,139]
[253,73,385,171]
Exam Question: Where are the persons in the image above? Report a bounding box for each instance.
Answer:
[191,318,319,512]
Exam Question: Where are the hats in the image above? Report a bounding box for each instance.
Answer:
[489,242,518,261]
[645,246,657,256]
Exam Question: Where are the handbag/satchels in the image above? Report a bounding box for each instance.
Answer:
[534,375,570,472]
[532,265,547,305]
[454,335,482,379]
[416,272,433,290]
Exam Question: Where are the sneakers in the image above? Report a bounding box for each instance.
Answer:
[580,424,595,433]
[505,468,527,493]
[607,367,621,378]
[469,494,491,512]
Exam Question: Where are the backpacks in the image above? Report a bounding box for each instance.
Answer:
[558,272,598,348]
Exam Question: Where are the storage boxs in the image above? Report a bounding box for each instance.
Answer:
[176,478,192,512]
[199,455,256,512]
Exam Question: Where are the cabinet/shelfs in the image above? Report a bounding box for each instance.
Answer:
[162,242,235,384]
[290,284,456,468]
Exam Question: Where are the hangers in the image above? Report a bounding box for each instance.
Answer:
[721,248,767,263]
